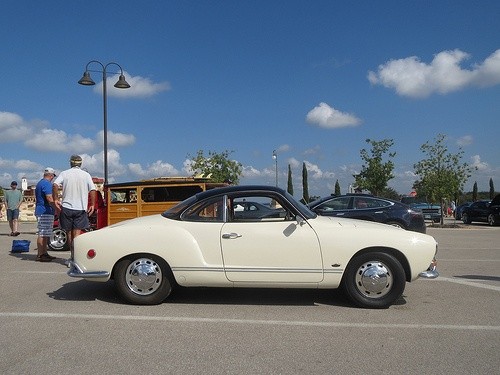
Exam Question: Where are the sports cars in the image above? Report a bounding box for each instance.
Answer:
[65,183,439,310]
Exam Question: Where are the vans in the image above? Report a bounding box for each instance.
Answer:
[45,174,235,251]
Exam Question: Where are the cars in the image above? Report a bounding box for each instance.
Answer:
[215,200,285,220]
[452,193,500,227]
[276,193,427,235]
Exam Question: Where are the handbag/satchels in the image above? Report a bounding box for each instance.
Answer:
[12,240,29,252]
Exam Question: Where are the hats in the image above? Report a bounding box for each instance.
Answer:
[11,181,17,187]
[44,167,56,177]
[224,179,232,183]
[71,155,82,163]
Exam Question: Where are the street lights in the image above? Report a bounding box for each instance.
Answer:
[77,60,131,185]
[271,149,278,187]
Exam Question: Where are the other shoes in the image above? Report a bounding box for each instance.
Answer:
[11,231,20,236]
[65,258,73,267]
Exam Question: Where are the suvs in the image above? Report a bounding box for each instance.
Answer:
[400,195,442,223]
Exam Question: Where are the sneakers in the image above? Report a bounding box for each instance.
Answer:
[40,254,51,262]
[45,253,56,259]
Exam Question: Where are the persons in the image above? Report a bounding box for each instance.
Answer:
[52,155,97,268]
[35,168,60,262]
[4,181,23,236]
